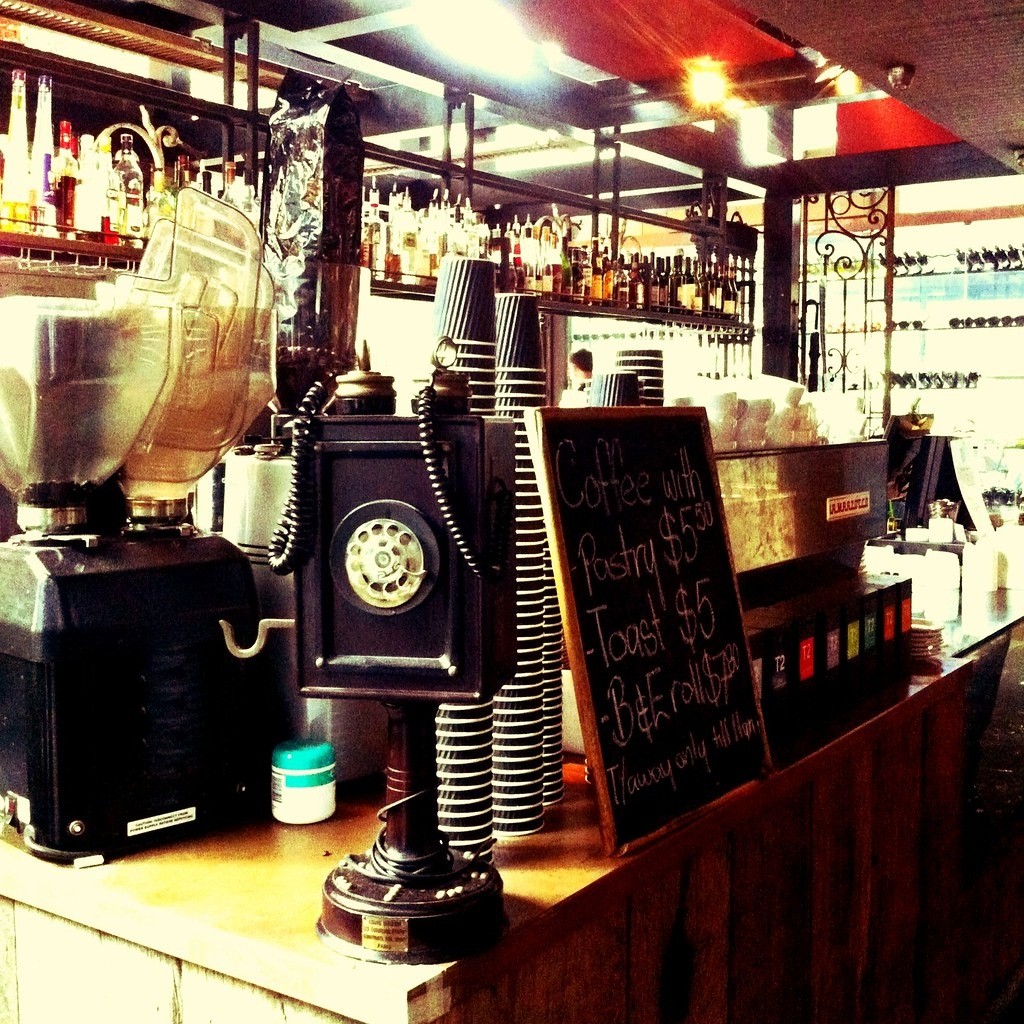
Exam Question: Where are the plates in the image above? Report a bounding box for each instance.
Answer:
[910,617,945,662]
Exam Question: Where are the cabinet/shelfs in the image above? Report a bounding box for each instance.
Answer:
[799,266,1024,391]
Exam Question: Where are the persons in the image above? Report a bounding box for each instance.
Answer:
[558,349,593,410]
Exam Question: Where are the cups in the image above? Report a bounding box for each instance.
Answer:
[989,513,1003,527]
[887,517,902,531]
[587,346,666,409]
[541,539,565,809]
[665,384,829,453]
[429,253,497,862]
[493,288,545,835]
[927,502,959,522]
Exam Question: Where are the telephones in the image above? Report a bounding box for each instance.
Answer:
[288,337,520,968]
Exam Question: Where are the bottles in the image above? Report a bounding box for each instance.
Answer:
[804,256,878,279]
[572,329,685,342]
[877,250,936,275]
[3,68,237,250]
[955,243,1024,272]
[492,219,741,322]
[360,175,490,278]
[879,370,978,389]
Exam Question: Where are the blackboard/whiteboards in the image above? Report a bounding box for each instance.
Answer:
[524,405,772,855]
